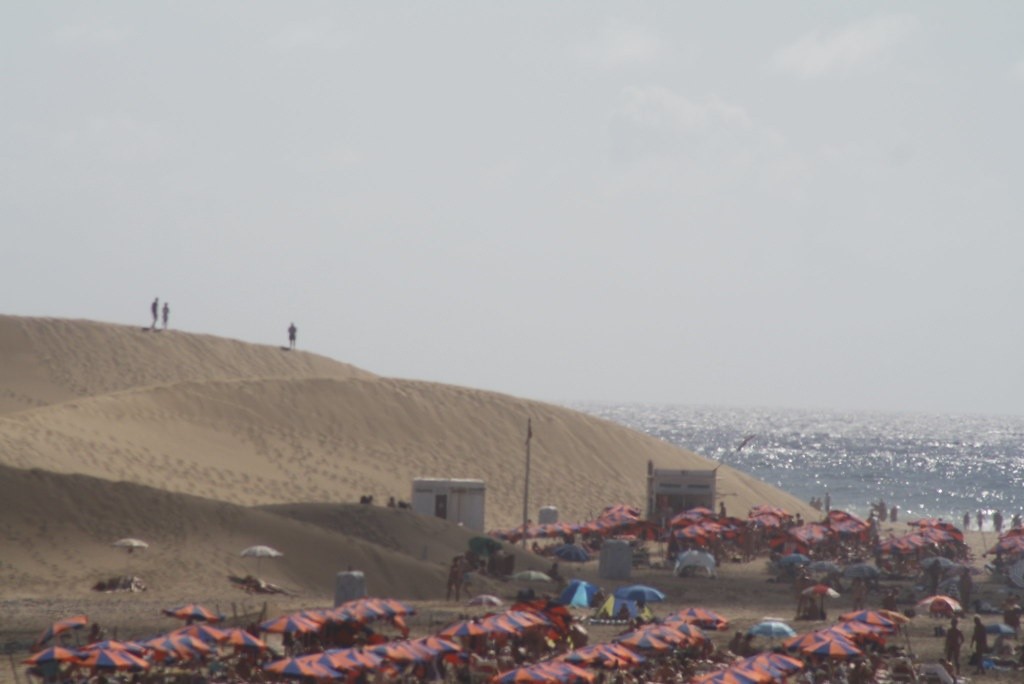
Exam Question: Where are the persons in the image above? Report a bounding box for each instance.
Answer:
[150,298,160,327]
[969,616,989,675]
[446,554,476,602]
[163,301,171,329]
[287,322,298,348]
[943,617,964,675]
[806,491,1024,533]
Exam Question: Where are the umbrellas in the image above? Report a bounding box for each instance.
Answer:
[241,545,283,576]
[486,504,1024,632]
[23,595,911,684]
[112,538,148,576]
